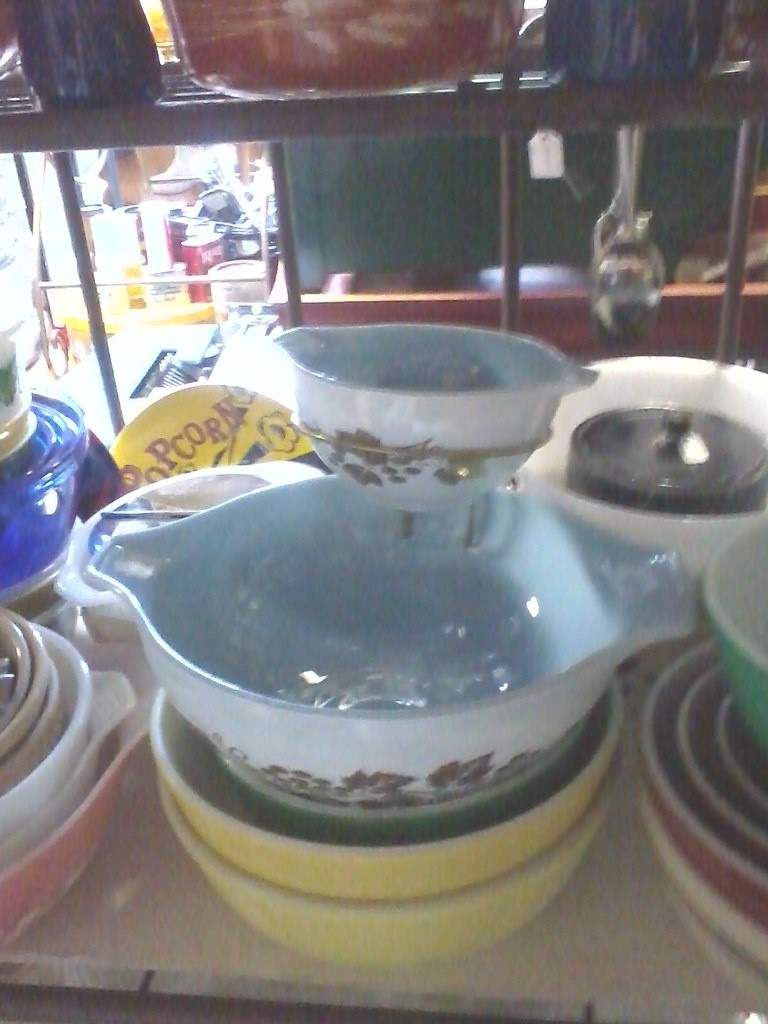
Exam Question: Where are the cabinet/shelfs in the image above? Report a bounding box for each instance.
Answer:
[0,57,768,1023]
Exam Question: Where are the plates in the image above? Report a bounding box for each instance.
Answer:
[89,475,271,562]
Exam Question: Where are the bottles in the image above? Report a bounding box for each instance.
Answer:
[11,0,164,115]
[163,1,524,100]
[544,1,728,89]
[590,214,662,356]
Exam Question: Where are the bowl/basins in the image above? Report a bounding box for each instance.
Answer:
[53,461,326,626]
[0,604,164,949]
[0,389,90,627]
[89,476,699,965]
[567,406,768,514]
[276,323,598,514]
[638,507,767,1004]
[518,355,768,574]
